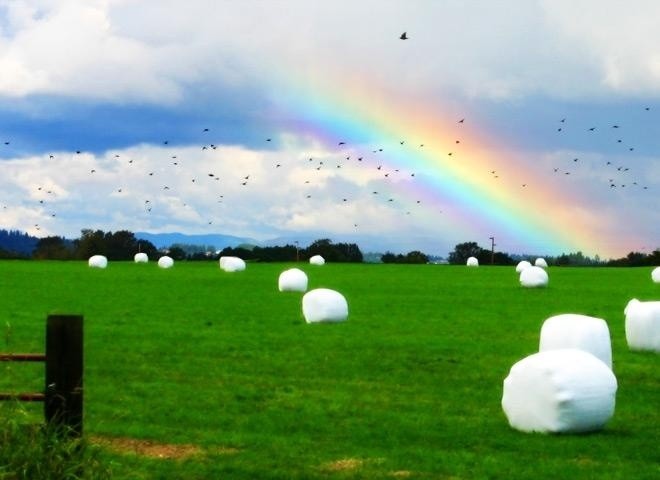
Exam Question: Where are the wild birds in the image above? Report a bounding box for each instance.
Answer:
[34,187,57,225]
[353,224,359,227]
[553,157,578,175]
[118,188,123,193]
[643,186,648,190]
[4,141,9,145]
[633,182,637,185]
[305,141,425,216]
[588,126,594,132]
[91,169,95,173]
[266,137,271,145]
[558,117,565,132]
[242,175,250,185]
[399,31,408,40]
[48,153,55,159]
[115,155,133,164]
[621,184,625,188]
[616,139,624,142]
[491,169,499,179]
[447,151,453,157]
[458,118,468,125]
[202,128,210,132]
[645,106,650,111]
[455,140,460,144]
[606,161,611,166]
[612,123,619,129]
[145,139,224,211]
[522,183,527,187]
[629,148,634,151]
[623,167,630,172]
[208,220,213,225]
[276,163,280,168]
[610,178,616,189]
[618,167,621,170]
[76,149,81,156]
